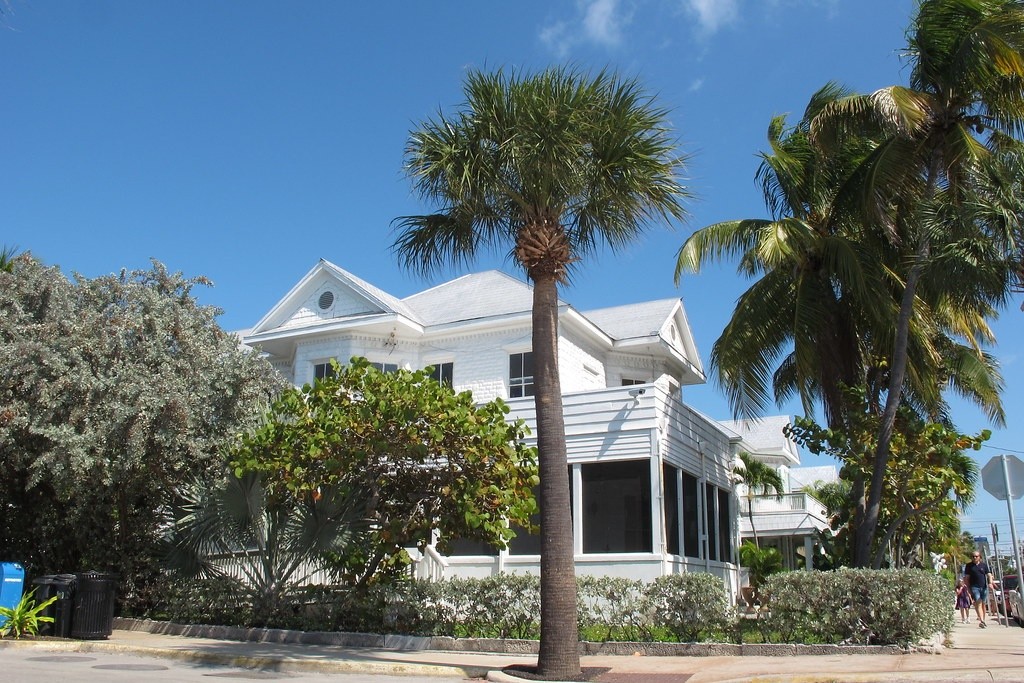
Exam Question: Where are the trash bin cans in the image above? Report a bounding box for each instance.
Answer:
[0,561,24,628]
[32,572,117,640]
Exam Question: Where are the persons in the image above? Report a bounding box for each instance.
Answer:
[955,577,974,624]
[964,550,994,628]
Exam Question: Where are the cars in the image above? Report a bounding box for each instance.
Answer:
[989,581,1003,605]
[1000,574,1024,616]
[1009,585,1024,628]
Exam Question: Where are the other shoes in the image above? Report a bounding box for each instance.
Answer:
[982,621,988,627]
[979,623,985,629]
